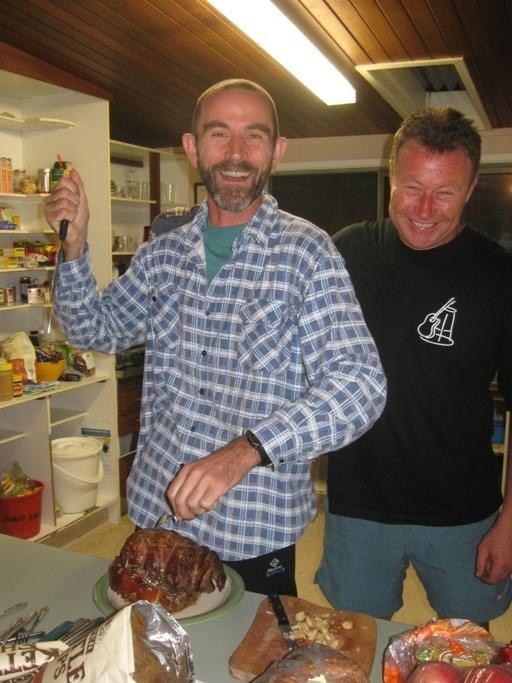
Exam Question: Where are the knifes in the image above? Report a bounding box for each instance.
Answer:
[45,220,68,333]
[267,591,299,653]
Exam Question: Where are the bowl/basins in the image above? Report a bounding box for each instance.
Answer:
[37,333,71,359]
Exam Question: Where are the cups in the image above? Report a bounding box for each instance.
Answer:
[135,175,148,199]
[163,183,175,203]
[120,235,133,252]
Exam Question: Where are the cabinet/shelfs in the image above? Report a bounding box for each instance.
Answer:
[0,46,116,409]
[0,374,122,549]
[110,139,193,379]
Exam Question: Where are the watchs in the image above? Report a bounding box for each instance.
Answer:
[244,428,273,468]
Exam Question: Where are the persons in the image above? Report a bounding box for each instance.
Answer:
[41,78,388,599]
[308,108,512,635]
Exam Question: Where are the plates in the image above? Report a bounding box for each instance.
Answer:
[92,563,245,627]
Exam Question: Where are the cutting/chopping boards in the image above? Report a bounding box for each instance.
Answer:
[228,595,377,681]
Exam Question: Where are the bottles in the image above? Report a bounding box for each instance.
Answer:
[12,375,23,398]
[19,277,31,302]
[0,363,13,402]
[5,287,14,303]
[8,283,16,301]
[0,157,12,193]
[12,168,26,192]
[37,167,51,192]
[0,289,7,305]
